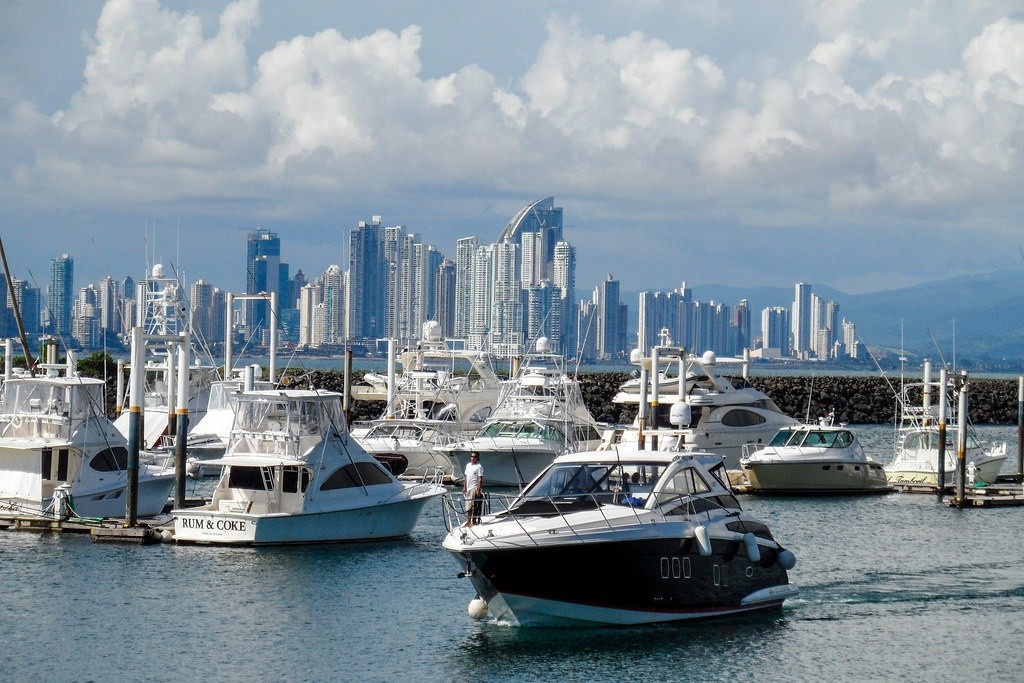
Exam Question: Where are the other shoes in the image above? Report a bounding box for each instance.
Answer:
[462,522,473,528]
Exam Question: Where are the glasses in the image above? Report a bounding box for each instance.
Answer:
[470,455,478,458]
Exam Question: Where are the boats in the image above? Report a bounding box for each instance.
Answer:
[0,218,607,545]
[595,318,1008,493]
[441,348,801,628]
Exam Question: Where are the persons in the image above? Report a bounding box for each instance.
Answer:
[462,451,483,528]
[923,420,931,449]
